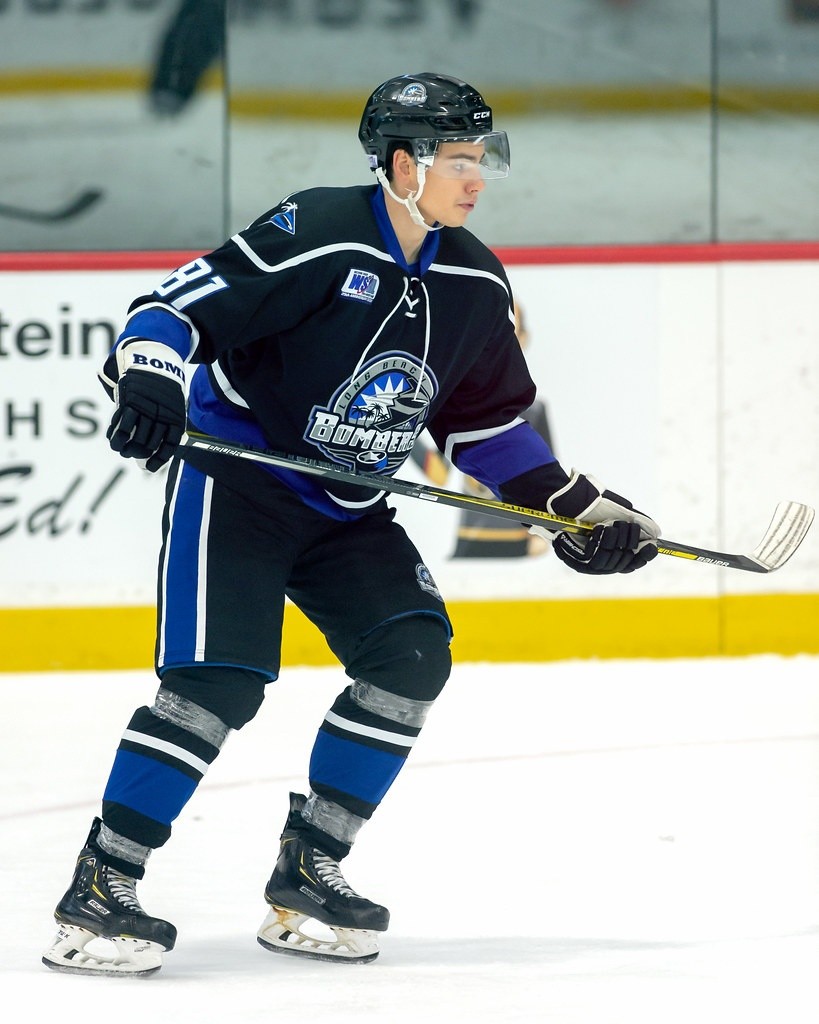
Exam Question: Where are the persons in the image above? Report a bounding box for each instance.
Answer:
[40,73,662,977]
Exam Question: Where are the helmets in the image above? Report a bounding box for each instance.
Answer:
[356,71,495,172]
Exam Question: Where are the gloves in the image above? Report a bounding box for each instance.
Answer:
[105,335,187,473]
[528,466,662,576]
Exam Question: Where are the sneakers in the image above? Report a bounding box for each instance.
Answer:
[256,789,391,966]
[39,814,178,977]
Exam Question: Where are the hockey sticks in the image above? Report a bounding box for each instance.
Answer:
[165,427,819,578]
